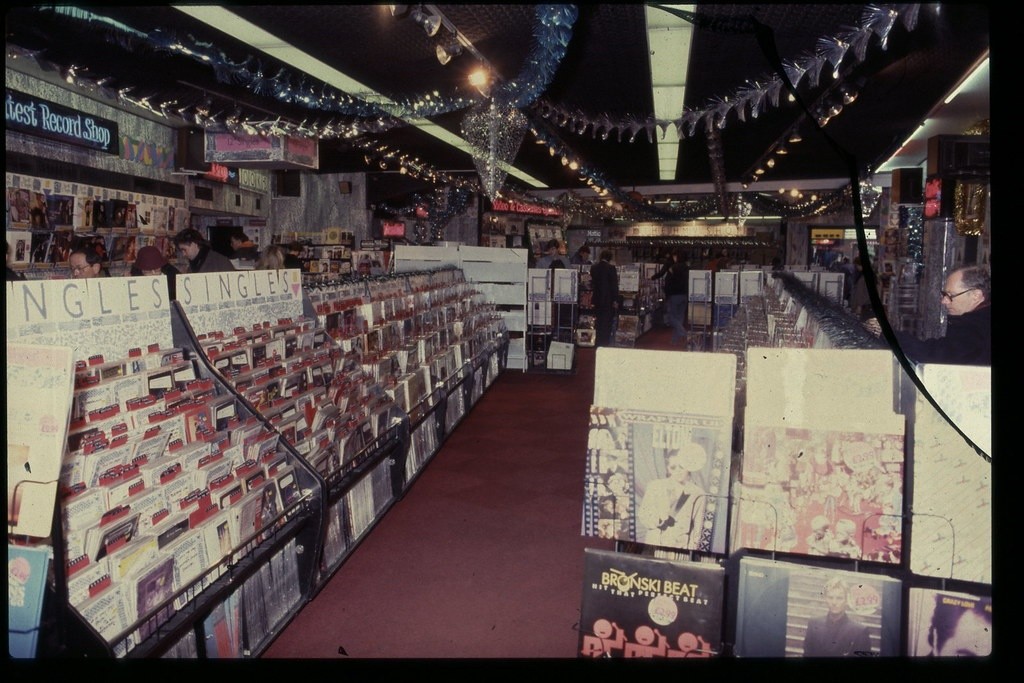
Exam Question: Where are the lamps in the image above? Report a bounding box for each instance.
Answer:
[410,4,441,37]
[840,83,859,105]
[389,5,408,16]
[529,125,611,197]
[815,107,830,128]
[436,29,462,66]
[742,126,802,189]
[827,97,842,117]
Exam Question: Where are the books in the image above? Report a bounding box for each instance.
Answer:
[8,185,511,658]
[512,225,991,658]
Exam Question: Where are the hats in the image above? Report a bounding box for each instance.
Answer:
[135,246,168,269]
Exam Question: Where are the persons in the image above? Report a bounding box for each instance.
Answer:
[283,241,309,272]
[230,230,260,260]
[571,246,592,265]
[174,227,235,273]
[131,246,183,301]
[879,263,896,300]
[865,263,991,364]
[254,245,286,270]
[69,246,103,278]
[850,256,872,315]
[668,245,691,346]
[924,592,991,658]
[829,257,854,300]
[802,575,872,657]
[638,449,706,552]
[648,242,679,327]
[536,239,572,269]
[823,239,843,268]
[720,248,731,258]
[590,250,619,347]
[713,256,738,273]
[702,253,725,272]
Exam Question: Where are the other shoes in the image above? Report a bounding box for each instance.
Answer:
[672,341,679,348]
[683,334,692,347]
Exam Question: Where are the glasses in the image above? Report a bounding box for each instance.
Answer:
[939,289,976,302]
[69,265,90,274]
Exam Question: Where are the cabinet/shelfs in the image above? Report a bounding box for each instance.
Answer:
[44,301,322,659]
[307,267,512,499]
[173,289,411,602]
